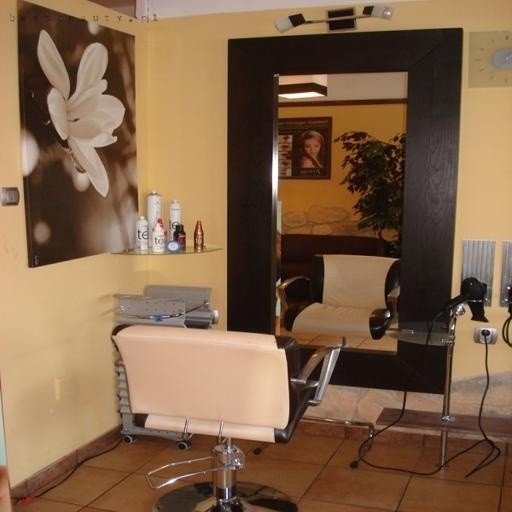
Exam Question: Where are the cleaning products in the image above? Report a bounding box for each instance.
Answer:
[135,191,204,254]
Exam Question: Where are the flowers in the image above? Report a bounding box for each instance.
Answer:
[26,28,126,198]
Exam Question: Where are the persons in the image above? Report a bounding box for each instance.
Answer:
[296,130,326,167]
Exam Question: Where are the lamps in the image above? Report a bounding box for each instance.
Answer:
[274,5,393,34]
[278,74,328,100]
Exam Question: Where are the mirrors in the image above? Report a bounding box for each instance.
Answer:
[226,25,463,393]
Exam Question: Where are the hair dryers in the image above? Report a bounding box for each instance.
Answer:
[442,277,488,323]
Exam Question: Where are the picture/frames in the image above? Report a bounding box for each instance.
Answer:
[278,116,332,180]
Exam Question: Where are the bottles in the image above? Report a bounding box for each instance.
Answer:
[136,190,204,253]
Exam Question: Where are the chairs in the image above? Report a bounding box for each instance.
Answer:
[112,323,342,512]
[279,253,400,345]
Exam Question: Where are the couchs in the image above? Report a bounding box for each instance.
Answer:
[281,235,390,300]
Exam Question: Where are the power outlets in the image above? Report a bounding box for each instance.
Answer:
[473,328,498,345]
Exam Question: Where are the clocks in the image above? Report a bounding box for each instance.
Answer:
[467,31,512,88]
